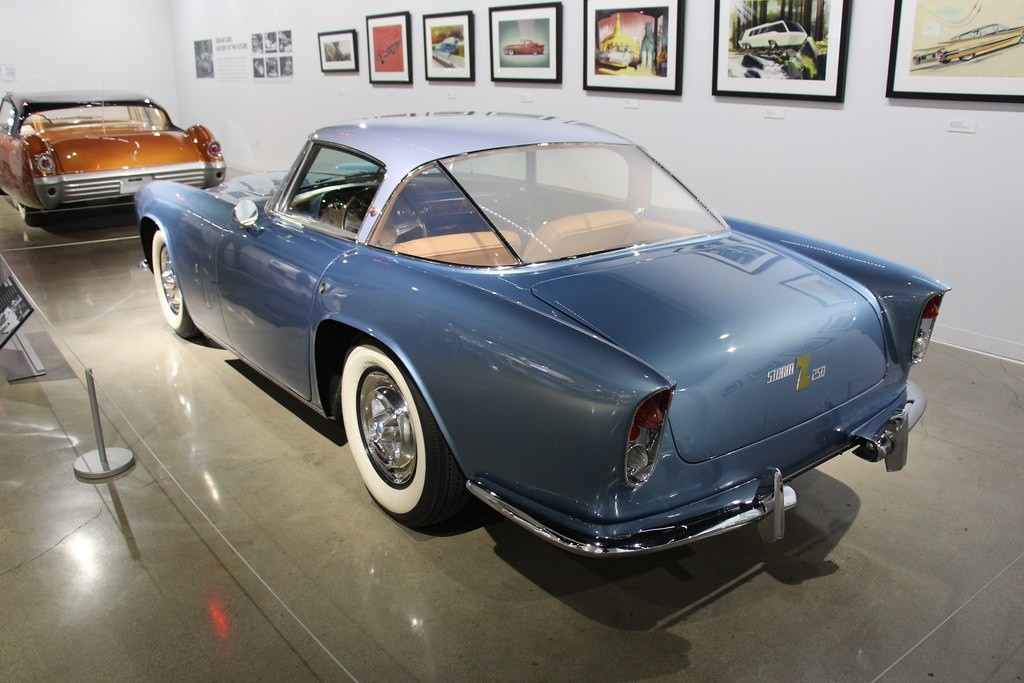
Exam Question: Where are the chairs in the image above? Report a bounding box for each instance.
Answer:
[392,228,523,268]
[522,209,643,261]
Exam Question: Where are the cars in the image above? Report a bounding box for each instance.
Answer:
[0,93,227,226]
[134,111,955,559]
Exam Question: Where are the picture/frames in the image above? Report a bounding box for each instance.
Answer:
[886,0,1024,104]
[582,0,685,96]
[712,0,853,103]
[422,9,475,81]
[488,2,563,83]
[317,29,359,73]
[365,11,413,86]
